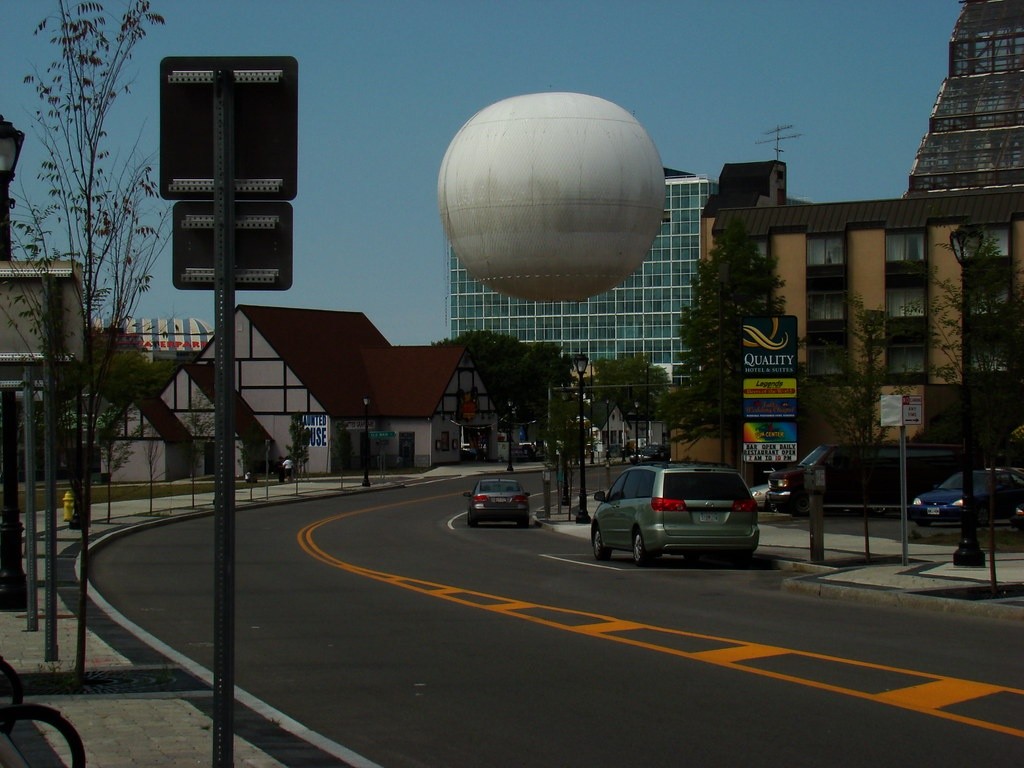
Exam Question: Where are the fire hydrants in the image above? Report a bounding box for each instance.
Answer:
[62,491,75,523]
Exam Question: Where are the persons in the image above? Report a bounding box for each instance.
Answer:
[276,456,293,485]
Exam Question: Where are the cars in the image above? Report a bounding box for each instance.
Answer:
[909,466,1024,528]
[628,445,672,466]
[750,484,778,512]
[463,476,531,528]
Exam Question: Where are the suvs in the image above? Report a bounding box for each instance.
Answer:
[591,461,758,566]
[770,442,964,518]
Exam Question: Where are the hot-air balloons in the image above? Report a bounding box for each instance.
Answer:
[437,92,667,344]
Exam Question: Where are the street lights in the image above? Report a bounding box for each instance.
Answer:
[573,354,591,524]
[950,219,986,568]
[634,400,641,464]
[507,399,514,470]
[605,396,611,459]
[362,393,371,487]
[561,382,570,506]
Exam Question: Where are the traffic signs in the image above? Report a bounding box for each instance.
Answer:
[368,432,397,437]
